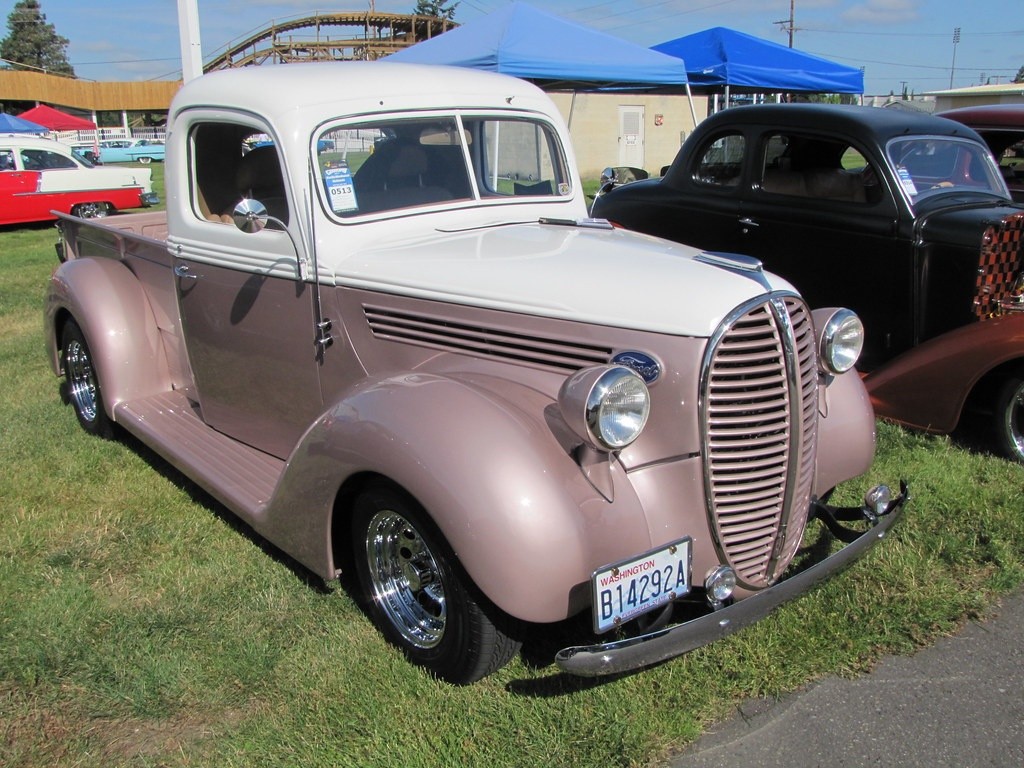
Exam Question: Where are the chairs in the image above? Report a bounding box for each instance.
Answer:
[222,146,289,231]
[808,168,866,204]
[358,140,454,213]
[999,166,1015,178]
[760,172,809,198]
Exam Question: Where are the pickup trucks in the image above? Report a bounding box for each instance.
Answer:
[42,59,912,687]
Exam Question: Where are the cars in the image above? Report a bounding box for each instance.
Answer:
[74,137,165,165]
[588,103,1023,464]
[1,136,160,227]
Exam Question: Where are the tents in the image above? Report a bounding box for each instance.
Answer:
[18,104,97,150]
[377,0,698,192]
[0,112,49,136]
[585,25,865,163]
